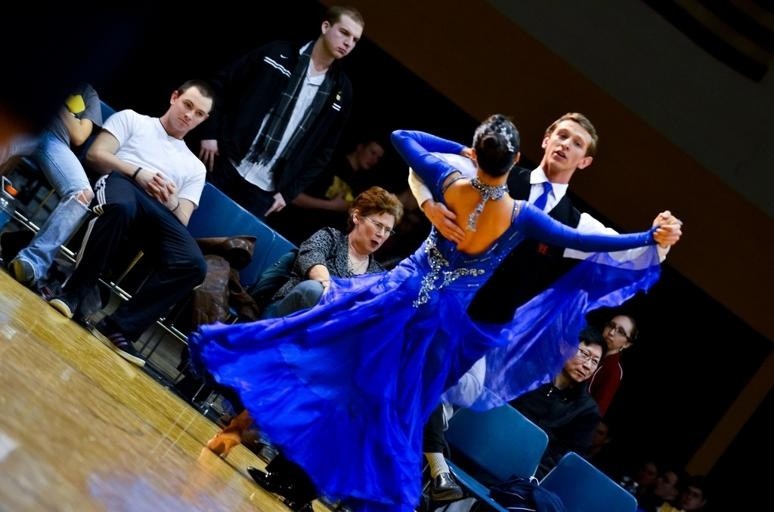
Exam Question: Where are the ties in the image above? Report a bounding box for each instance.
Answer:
[531,177,554,213]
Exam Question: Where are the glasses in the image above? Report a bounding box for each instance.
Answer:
[365,217,395,240]
[579,344,601,373]
[608,324,631,342]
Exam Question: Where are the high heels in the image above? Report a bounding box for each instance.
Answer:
[208,432,240,457]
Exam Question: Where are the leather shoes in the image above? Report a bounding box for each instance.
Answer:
[247,465,314,512]
[427,470,464,500]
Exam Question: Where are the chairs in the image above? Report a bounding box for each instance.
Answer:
[2,98,642,511]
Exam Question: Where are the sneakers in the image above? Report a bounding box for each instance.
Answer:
[91,314,145,370]
[49,284,78,320]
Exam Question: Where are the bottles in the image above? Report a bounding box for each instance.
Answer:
[619,475,628,488]
[629,481,638,494]
[0,183,17,229]
